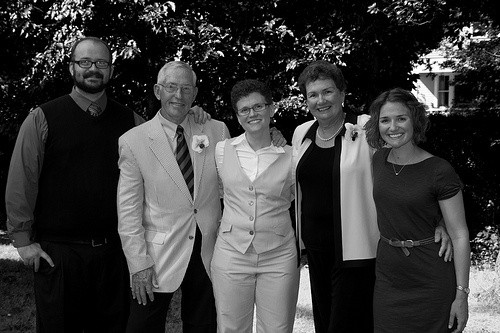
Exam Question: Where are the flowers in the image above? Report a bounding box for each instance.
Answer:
[345,123,362,140]
[192,135,210,153]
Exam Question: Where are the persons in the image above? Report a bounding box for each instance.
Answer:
[210,79,300,333]
[365,90,471,333]
[292,61,453,333]
[6,38,211,333]
[117,61,287,333]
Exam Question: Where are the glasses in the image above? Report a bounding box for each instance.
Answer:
[72,60,110,69]
[236,103,271,116]
[158,83,193,94]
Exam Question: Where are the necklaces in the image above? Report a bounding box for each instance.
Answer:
[317,121,344,141]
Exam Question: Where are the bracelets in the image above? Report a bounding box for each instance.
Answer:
[390,149,414,175]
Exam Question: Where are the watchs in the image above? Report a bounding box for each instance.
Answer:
[456,286,470,293]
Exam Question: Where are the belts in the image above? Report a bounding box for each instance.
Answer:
[380,234,435,257]
[58,233,120,247]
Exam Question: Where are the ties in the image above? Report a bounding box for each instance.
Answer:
[87,103,102,117]
[176,125,194,201]
